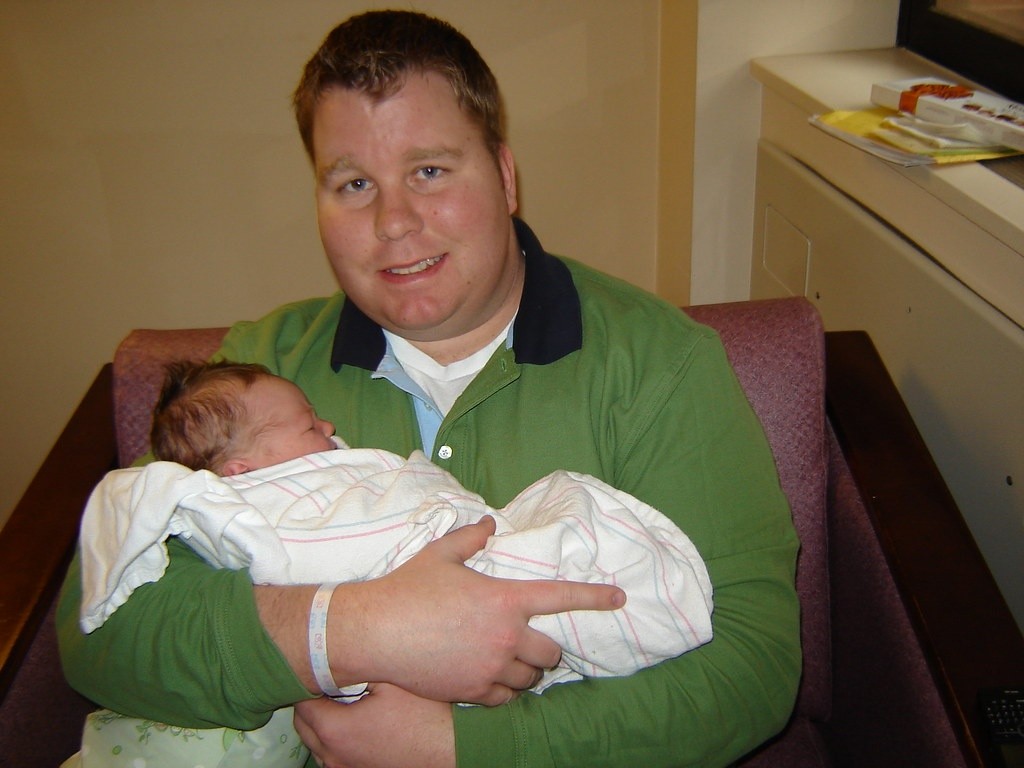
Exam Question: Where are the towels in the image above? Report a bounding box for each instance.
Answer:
[77,436,713,705]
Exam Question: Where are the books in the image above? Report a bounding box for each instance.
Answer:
[805,107,1021,170]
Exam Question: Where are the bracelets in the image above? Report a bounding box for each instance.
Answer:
[309,584,368,697]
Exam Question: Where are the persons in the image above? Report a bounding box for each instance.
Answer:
[55,11,803,768]
[150,356,714,678]
